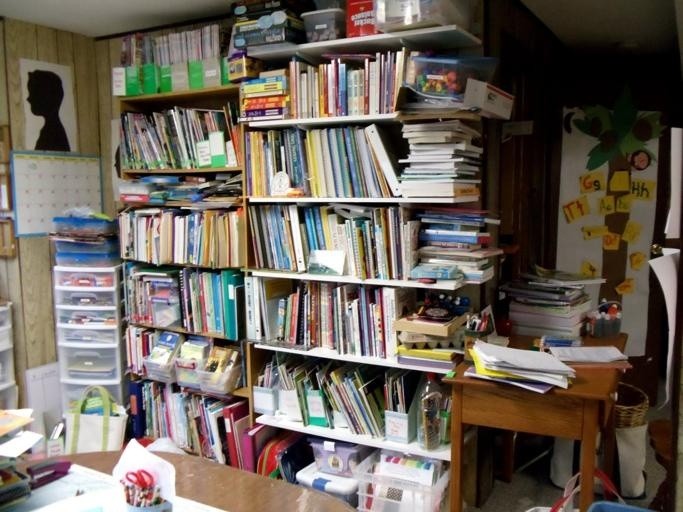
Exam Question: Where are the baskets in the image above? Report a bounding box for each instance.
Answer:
[611,380,652,427]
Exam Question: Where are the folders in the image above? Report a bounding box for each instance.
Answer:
[365,122,403,197]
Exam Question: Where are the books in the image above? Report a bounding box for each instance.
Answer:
[464,265,629,394]
[120,17,505,470]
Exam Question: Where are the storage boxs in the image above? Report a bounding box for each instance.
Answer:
[53,267,129,411]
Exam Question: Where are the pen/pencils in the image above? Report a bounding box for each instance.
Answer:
[124,485,162,508]
[465,311,491,331]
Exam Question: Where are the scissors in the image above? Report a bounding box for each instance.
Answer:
[125,470,154,489]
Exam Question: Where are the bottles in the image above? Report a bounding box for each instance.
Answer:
[417,372,442,452]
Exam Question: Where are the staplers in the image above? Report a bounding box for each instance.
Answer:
[26,459,72,488]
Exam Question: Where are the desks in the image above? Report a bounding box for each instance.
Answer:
[442,333,631,512]
[16,451,362,511]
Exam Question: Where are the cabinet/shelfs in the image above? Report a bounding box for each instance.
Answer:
[110,24,251,477]
[243,21,505,511]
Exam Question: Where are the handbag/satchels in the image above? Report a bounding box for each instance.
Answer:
[64,385,130,455]
[550,421,649,500]
